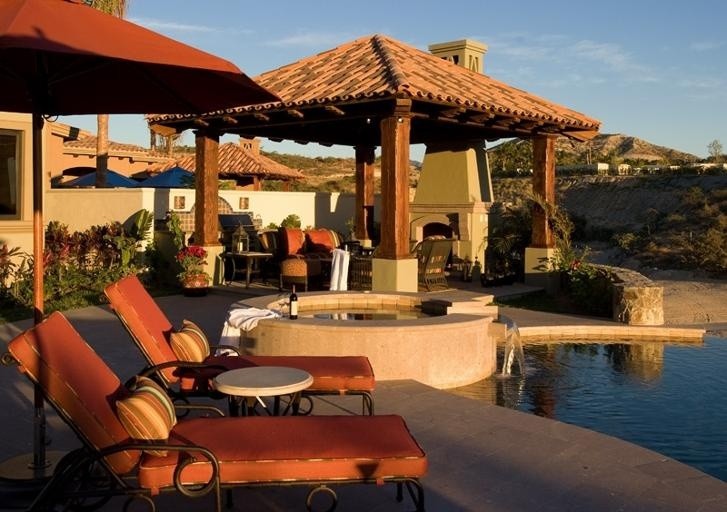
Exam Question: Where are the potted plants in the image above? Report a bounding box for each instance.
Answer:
[144,238,173,296]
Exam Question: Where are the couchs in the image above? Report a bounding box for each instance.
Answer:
[258,227,364,292]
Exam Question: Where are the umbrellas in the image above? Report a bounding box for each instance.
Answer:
[137,163,200,189]
[1,0,284,484]
[66,166,139,186]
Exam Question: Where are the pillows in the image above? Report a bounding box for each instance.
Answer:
[279,228,304,257]
[115,374,178,457]
[169,320,211,363]
[306,229,338,252]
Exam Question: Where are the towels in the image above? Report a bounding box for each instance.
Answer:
[215,306,278,355]
[330,249,349,291]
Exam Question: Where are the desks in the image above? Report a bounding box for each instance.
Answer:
[226,251,273,289]
[212,367,314,416]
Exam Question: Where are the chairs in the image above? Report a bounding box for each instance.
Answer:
[0,311,430,512]
[411,235,457,290]
[102,274,376,416]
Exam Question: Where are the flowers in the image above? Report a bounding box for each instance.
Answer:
[162,209,186,250]
[175,246,214,272]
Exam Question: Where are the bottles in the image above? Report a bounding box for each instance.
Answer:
[290,285,297,320]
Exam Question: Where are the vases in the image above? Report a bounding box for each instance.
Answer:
[182,274,211,297]
